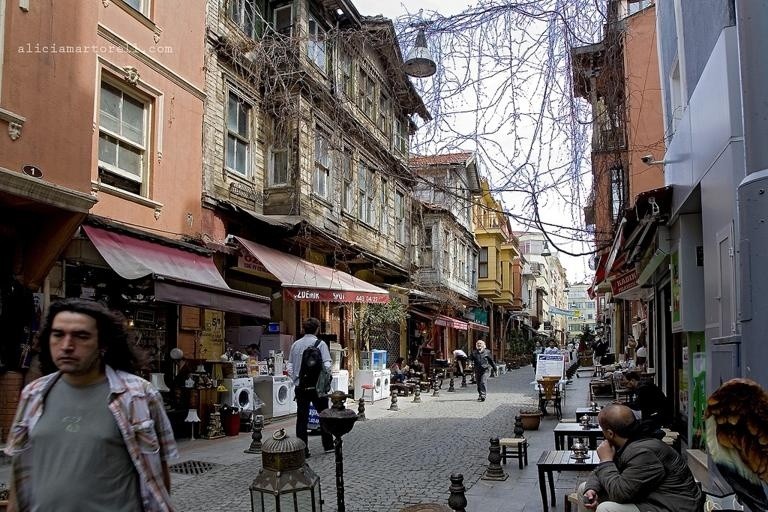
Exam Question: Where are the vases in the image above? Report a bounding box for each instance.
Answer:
[520,410,543,430]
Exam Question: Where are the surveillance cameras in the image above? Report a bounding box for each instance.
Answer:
[640,153,654,167]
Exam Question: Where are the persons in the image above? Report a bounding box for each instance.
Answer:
[570,402,705,512]
[588,335,605,376]
[389,356,410,397]
[460,338,498,402]
[450,347,468,380]
[408,358,425,396]
[612,368,673,429]
[0,294,182,512]
[284,317,338,459]
[527,339,586,380]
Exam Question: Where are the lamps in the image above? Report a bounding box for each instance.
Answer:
[184,408,203,441]
[170,348,184,368]
[149,373,170,405]
[403,26,436,77]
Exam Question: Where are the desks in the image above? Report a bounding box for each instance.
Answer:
[536,371,641,512]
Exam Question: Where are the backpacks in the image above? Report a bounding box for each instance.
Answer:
[294,339,323,389]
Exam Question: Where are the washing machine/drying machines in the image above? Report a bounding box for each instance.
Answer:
[221,370,348,418]
[354,370,390,401]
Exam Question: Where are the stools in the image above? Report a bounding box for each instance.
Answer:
[499,437,528,469]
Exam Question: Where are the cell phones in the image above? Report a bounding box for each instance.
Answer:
[578,491,593,503]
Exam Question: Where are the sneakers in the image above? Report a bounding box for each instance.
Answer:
[477,396,486,402]
[324,446,335,454]
